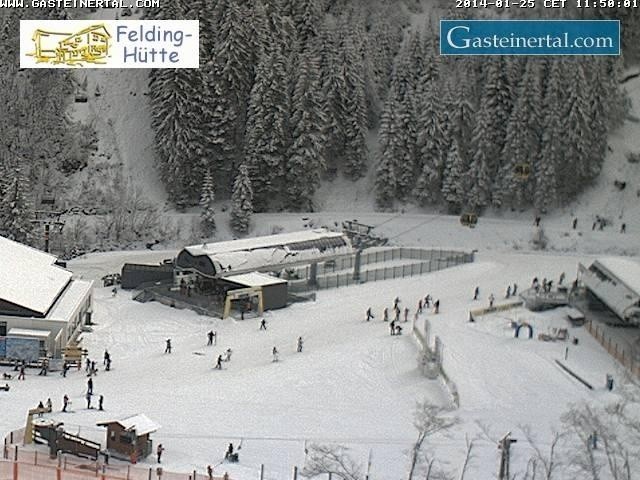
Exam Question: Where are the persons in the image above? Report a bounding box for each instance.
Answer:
[547,278,553,292]
[259,318,268,330]
[473,286,480,301]
[559,271,567,285]
[533,276,538,291]
[542,277,548,291]
[572,217,578,231]
[206,330,215,347]
[225,443,234,461]
[271,345,279,363]
[156,444,165,464]
[621,223,626,234]
[534,213,541,228]
[164,339,172,355]
[215,354,225,370]
[505,285,511,299]
[223,348,233,364]
[488,292,496,309]
[297,334,304,353]
[365,291,440,335]
[12,349,112,418]
[513,283,518,297]
